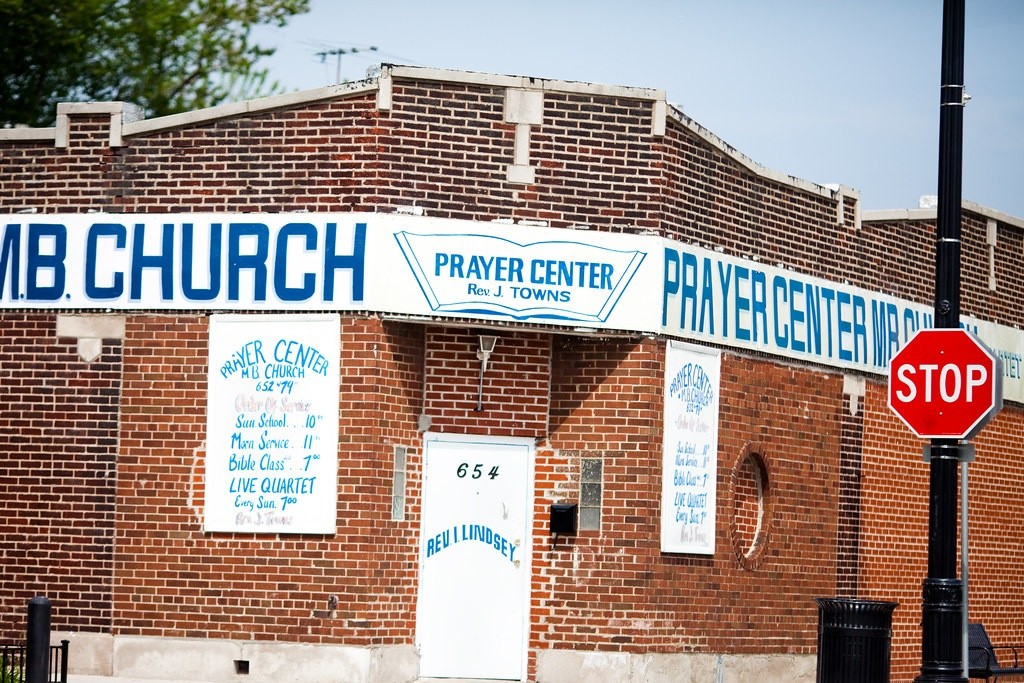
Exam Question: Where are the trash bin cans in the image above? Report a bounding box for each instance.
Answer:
[812,597,902,683]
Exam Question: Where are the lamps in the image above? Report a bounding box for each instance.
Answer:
[474,335,500,411]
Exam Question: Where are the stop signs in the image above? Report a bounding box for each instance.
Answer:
[888,328,1003,441]
